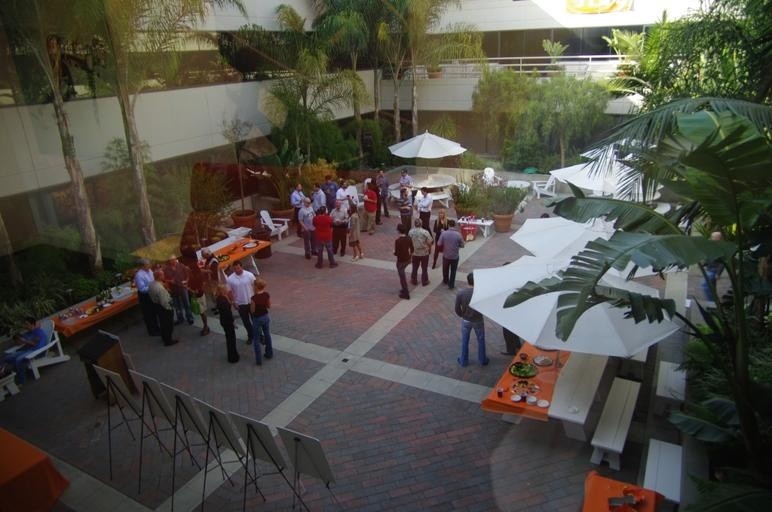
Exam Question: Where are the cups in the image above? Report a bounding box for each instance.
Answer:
[527,396,537,405]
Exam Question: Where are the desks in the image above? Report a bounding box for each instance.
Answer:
[0,428,50,512]
[409,174,457,189]
[457,218,494,238]
[51,235,272,337]
[478,341,666,512]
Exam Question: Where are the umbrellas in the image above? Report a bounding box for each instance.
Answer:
[388,128,468,179]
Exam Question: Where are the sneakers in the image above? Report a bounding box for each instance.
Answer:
[306,248,363,269]
[199,326,209,336]
[148,319,194,347]
[399,289,410,299]
[410,277,430,286]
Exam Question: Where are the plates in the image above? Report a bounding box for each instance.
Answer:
[242,242,257,249]
[510,394,521,402]
[533,355,553,366]
[537,399,549,408]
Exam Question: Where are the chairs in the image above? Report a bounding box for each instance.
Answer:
[5,318,71,380]
[259,209,291,241]
[482,167,556,199]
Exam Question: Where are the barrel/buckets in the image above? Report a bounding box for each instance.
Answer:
[460,222,478,241]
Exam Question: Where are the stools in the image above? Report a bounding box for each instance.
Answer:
[388,182,469,209]
[0,369,20,402]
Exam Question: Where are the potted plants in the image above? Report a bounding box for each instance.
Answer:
[542,39,570,77]
[427,66,443,79]
[218,116,258,229]
[446,182,530,233]
[242,136,305,225]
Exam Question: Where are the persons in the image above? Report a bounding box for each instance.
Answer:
[285,166,468,301]
[0,318,47,385]
[500,261,522,356]
[700,231,725,301]
[455,271,490,370]
[129,244,273,365]
[541,213,550,218]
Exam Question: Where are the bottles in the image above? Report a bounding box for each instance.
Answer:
[96,288,114,308]
[496,383,502,398]
